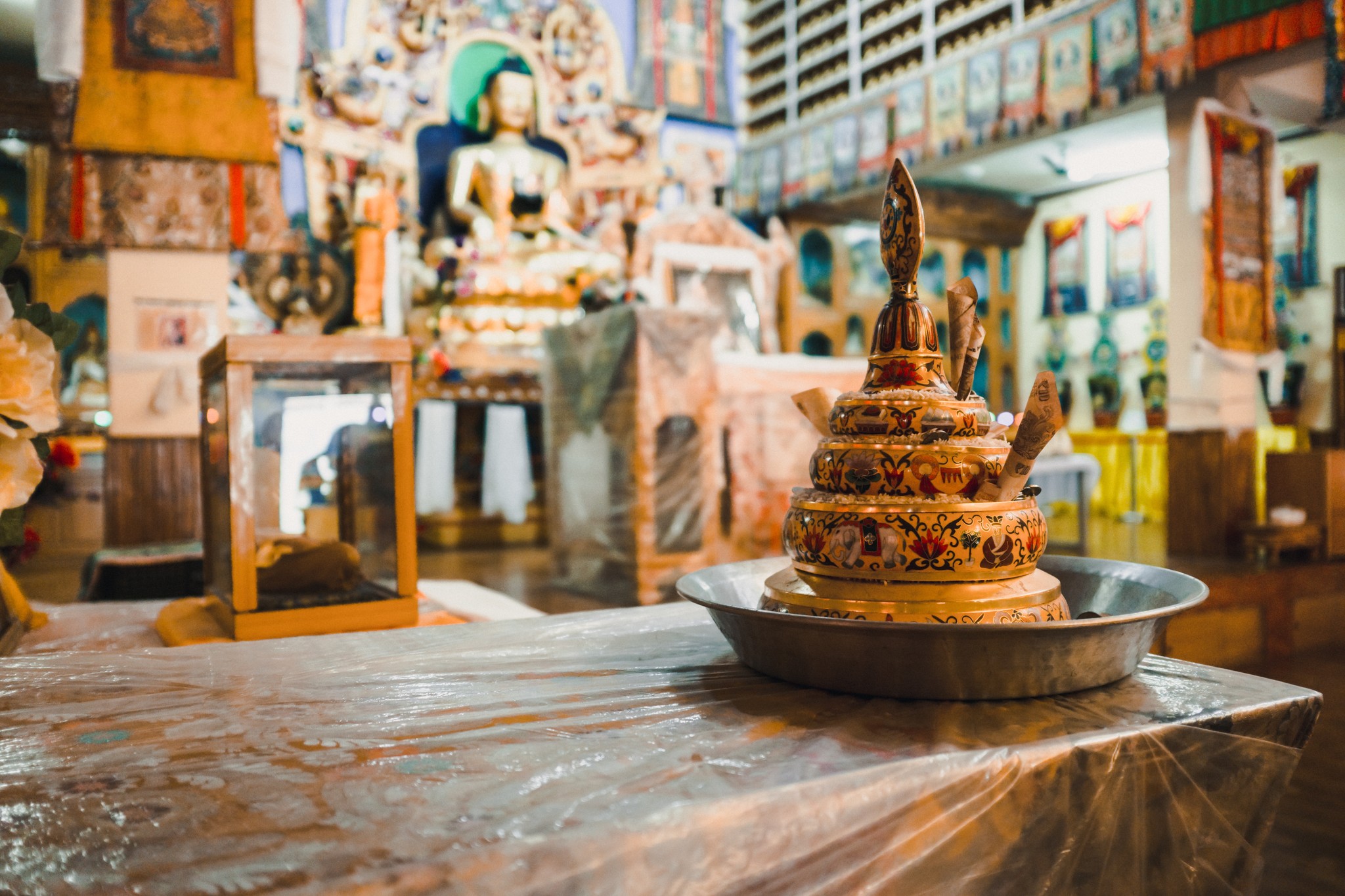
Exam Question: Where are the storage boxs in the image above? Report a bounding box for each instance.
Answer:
[198,334,420,641]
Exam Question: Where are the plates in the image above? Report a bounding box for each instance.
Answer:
[675,553,1211,700]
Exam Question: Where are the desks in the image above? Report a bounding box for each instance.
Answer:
[0,604,1325,896]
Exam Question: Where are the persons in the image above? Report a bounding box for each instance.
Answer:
[440,55,575,258]
